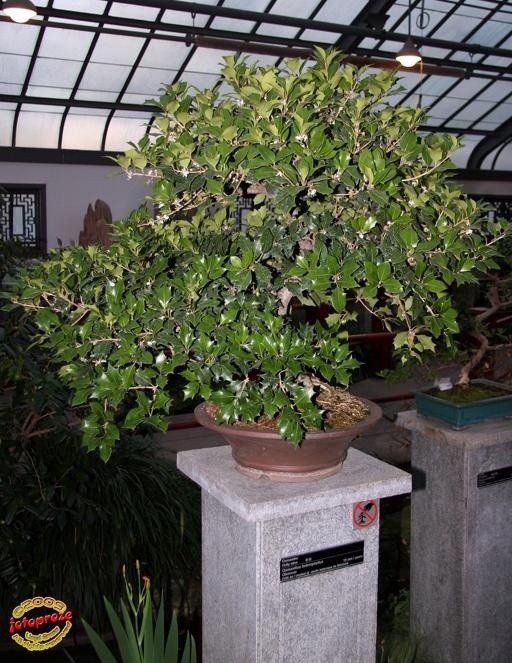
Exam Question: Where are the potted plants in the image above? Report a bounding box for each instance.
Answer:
[1,53,511,482]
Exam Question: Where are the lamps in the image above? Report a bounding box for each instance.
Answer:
[394,0,422,67]
